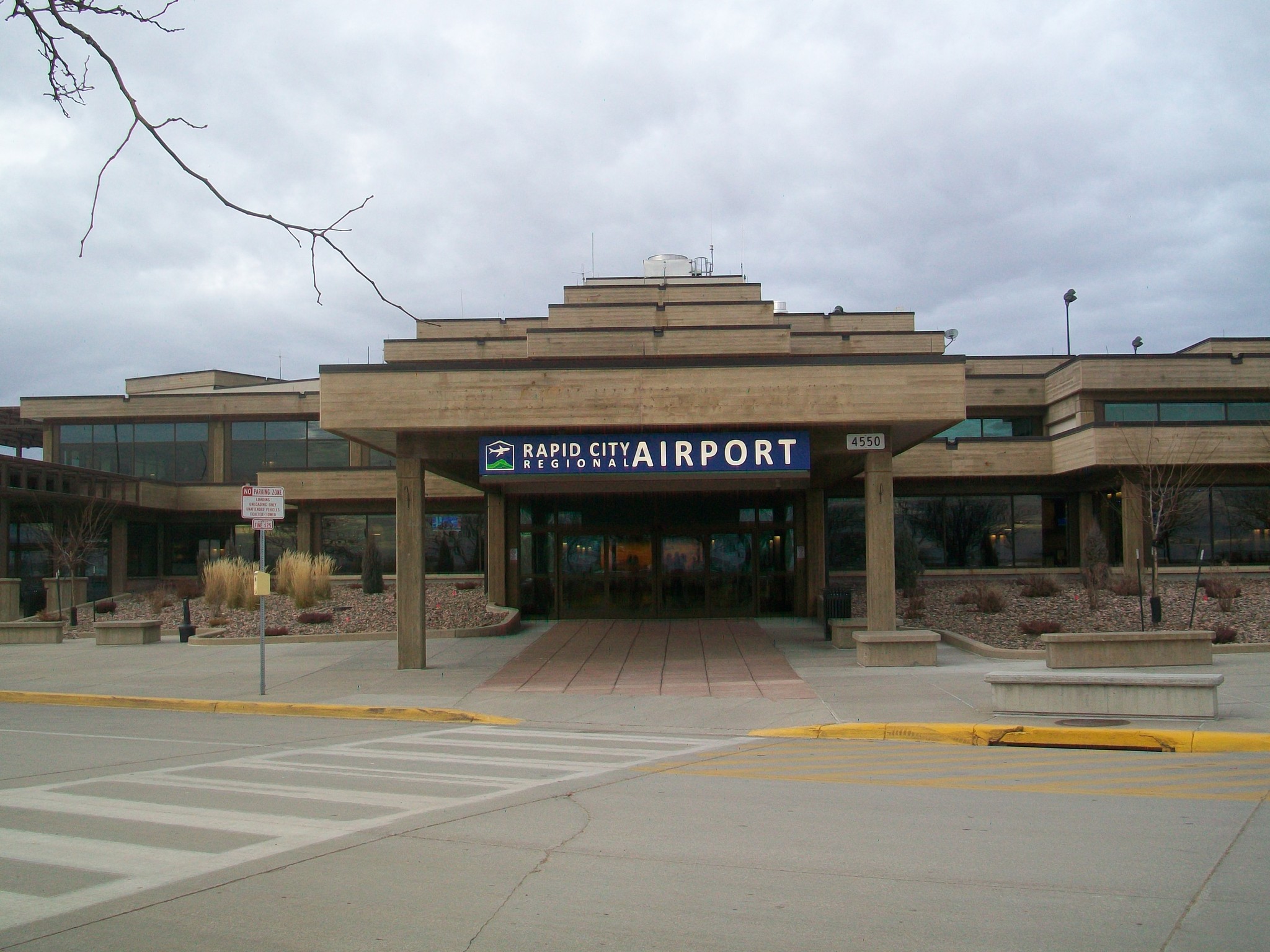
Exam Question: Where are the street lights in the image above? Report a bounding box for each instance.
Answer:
[1063,289,1077,356]
[1132,336,1148,355]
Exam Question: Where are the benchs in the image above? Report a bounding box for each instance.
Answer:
[828,616,903,649]
[1040,629,1217,666]
[850,630,940,668]
[94,620,164,644]
[984,672,1224,717]
[0,621,67,643]
